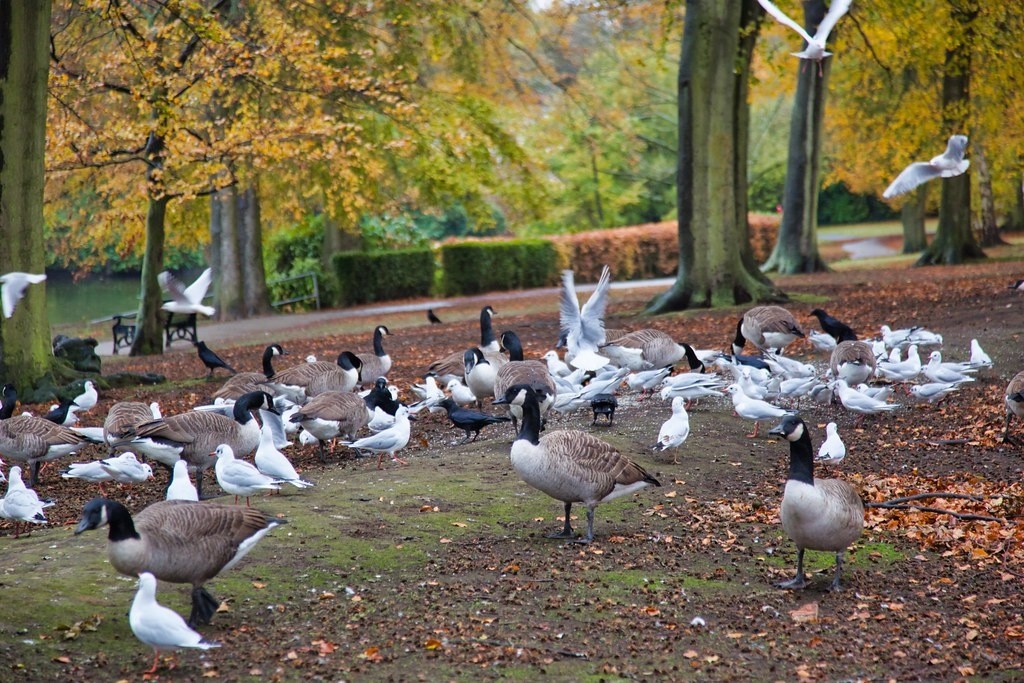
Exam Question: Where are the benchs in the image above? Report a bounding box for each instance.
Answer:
[113,302,197,353]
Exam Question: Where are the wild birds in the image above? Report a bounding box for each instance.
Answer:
[75,497,292,632]
[767,416,866,592]
[757,0,852,78]
[0,264,1024,545]
[882,135,970,200]
[130,571,222,674]
